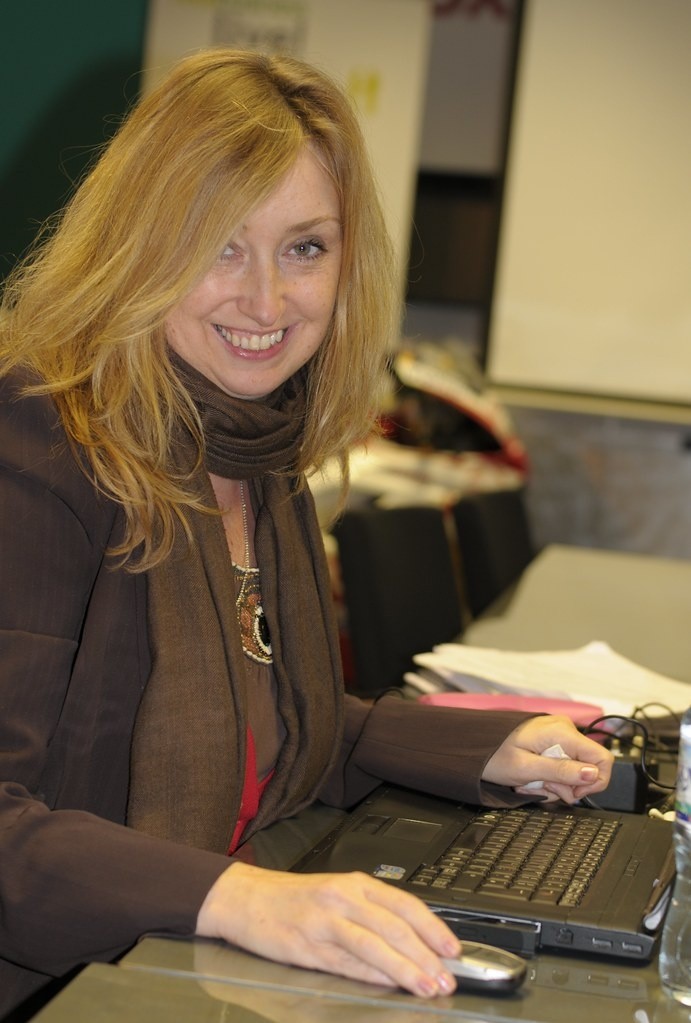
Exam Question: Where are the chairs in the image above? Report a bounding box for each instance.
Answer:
[330,506,460,702]
[451,490,535,624]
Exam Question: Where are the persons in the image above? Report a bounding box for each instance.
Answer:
[0,49,615,1023]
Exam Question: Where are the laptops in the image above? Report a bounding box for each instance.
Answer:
[283,776,678,956]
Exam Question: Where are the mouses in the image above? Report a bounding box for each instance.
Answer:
[439,939,528,993]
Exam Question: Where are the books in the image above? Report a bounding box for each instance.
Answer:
[403,639,691,737]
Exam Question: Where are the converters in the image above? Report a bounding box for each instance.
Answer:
[573,760,659,812]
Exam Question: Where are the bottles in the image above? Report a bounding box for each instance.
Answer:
[658,705,691,1008]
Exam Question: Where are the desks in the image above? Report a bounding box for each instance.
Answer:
[28,540,691,1023]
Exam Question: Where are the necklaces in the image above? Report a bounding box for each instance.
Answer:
[235,482,249,604]
